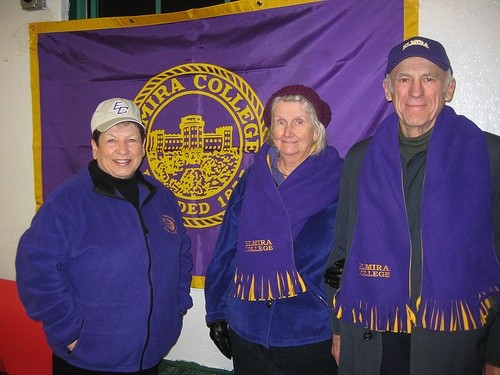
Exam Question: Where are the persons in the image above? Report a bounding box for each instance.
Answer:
[204,85,345,375]
[15,98,193,375]
[322,36,500,375]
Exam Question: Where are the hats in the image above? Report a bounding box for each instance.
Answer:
[263,84,332,128]
[91,98,146,136]
[385,37,453,77]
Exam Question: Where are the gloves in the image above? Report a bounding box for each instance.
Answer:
[324,259,345,288]
[208,321,233,359]
